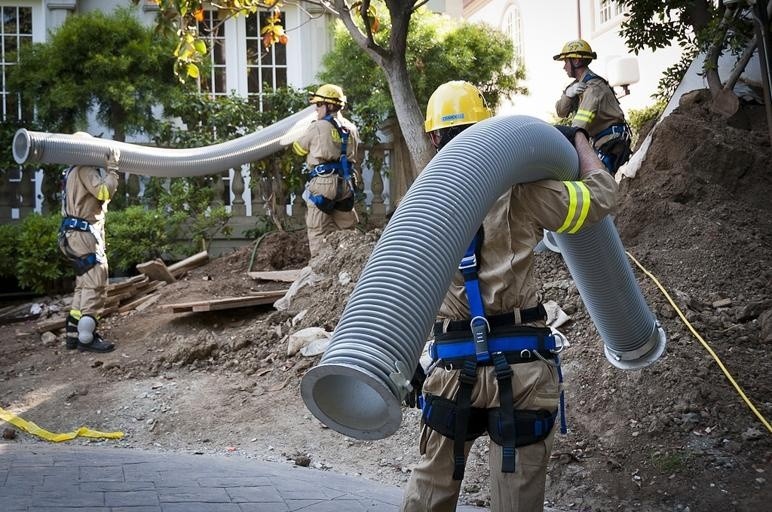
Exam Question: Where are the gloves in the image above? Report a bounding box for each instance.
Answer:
[552,124,590,149]
[104,146,121,171]
[565,82,587,99]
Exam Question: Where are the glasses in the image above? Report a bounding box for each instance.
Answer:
[316,101,330,107]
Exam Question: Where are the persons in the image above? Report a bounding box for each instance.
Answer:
[56,130,117,353]
[552,40,634,174]
[289,85,359,258]
[399,80,619,512]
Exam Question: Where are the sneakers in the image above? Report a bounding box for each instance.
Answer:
[76,333,116,353]
[66,336,79,350]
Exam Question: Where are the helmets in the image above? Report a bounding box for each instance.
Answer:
[555,39,597,61]
[308,84,346,107]
[424,80,493,134]
